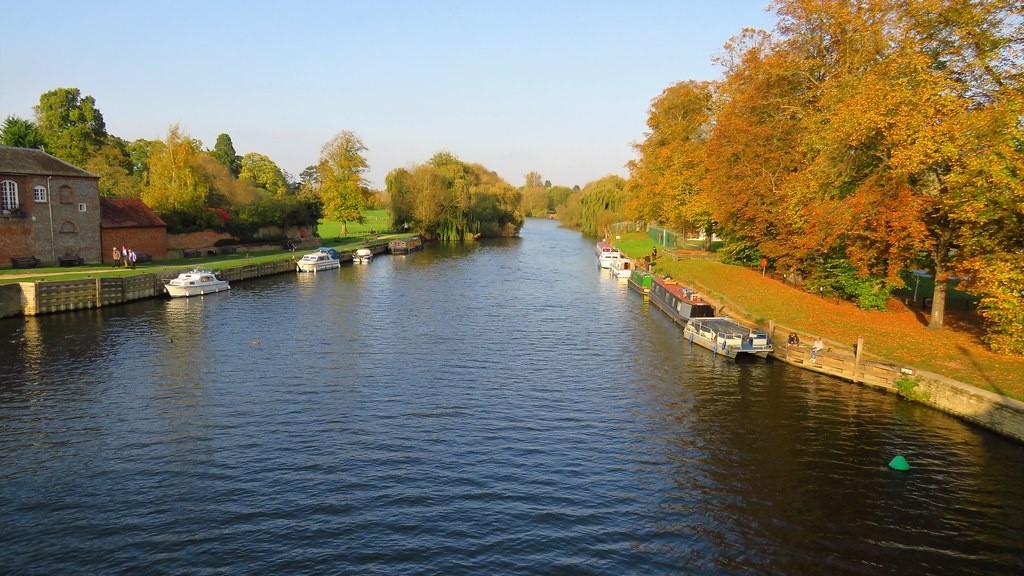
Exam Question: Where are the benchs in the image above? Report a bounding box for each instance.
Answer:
[58,255,85,267]
[183,249,202,258]
[11,255,41,269]
[136,255,152,263]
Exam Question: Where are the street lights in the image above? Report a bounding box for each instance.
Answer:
[47,176,57,264]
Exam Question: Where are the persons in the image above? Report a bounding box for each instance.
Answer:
[653,246,657,258]
[810,337,823,363]
[112,247,120,267]
[396,221,409,233]
[787,332,799,344]
[287,239,296,252]
[127,249,135,267]
[643,253,650,262]
[120,244,129,267]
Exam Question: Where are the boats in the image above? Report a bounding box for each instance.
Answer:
[649,275,715,327]
[388,237,423,255]
[609,258,636,278]
[162,267,231,297]
[352,248,373,263]
[290,250,341,274]
[595,242,621,269]
[628,268,660,301]
[682,316,774,362]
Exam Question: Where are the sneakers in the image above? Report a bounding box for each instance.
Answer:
[810,358,816,363]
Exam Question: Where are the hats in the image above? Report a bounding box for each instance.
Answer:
[120,244,127,249]
